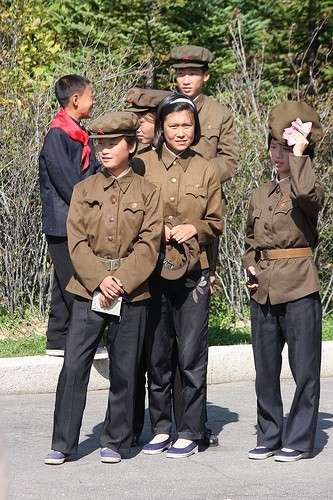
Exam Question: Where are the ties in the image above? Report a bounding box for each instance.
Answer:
[49,107,91,171]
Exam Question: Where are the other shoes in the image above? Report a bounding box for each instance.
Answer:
[195,427,219,446]
[167,438,199,458]
[100,447,121,463]
[142,435,174,454]
[273,446,310,462]
[45,349,65,356]
[248,446,281,459]
[92,346,109,360]
[43,451,66,464]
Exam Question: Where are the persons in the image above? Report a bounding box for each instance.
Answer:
[127,45,240,446]
[141,96,224,460]
[243,98,326,462]
[41,106,168,465]
[38,74,113,361]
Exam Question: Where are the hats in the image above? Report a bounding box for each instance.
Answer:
[268,99,323,146]
[122,88,173,114]
[87,110,140,138]
[151,215,200,281]
[168,44,213,69]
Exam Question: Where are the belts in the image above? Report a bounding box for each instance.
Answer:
[97,256,128,271]
[260,248,314,260]
